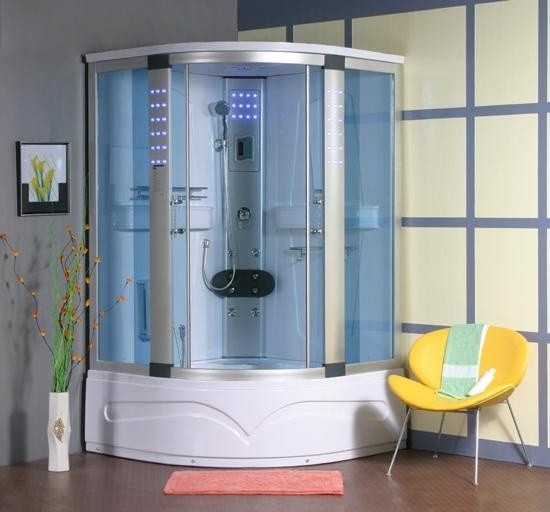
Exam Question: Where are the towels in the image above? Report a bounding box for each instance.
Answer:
[438,323,486,399]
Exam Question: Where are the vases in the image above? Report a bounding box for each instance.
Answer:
[47,393,72,471]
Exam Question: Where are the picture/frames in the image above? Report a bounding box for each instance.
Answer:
[16,141,73,216]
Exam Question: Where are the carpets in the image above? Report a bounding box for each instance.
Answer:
[163,470,344,495]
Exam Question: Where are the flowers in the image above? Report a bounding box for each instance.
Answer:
[2,231,133,393]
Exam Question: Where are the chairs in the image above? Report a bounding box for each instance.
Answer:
[388,324,531,487]
